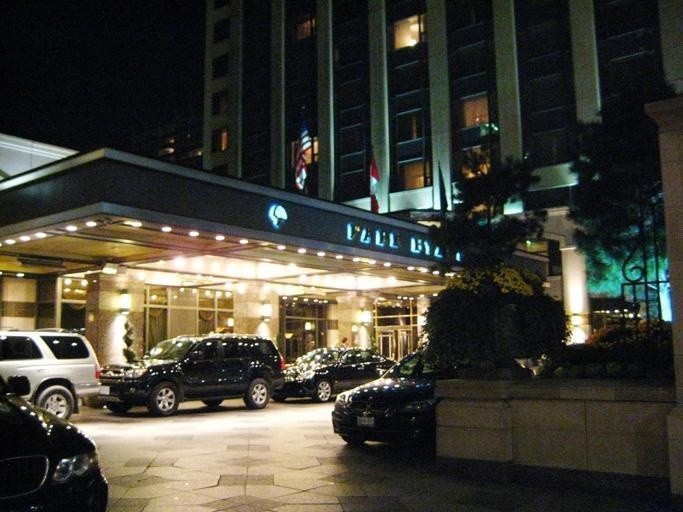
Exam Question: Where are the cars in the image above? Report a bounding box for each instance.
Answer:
[333,351,534,446]
[0,371,109,508]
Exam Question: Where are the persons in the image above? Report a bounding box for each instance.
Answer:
[341,337,348,348]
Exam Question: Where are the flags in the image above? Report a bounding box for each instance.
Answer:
[292,117,314,191]
[368,156,382,214]
